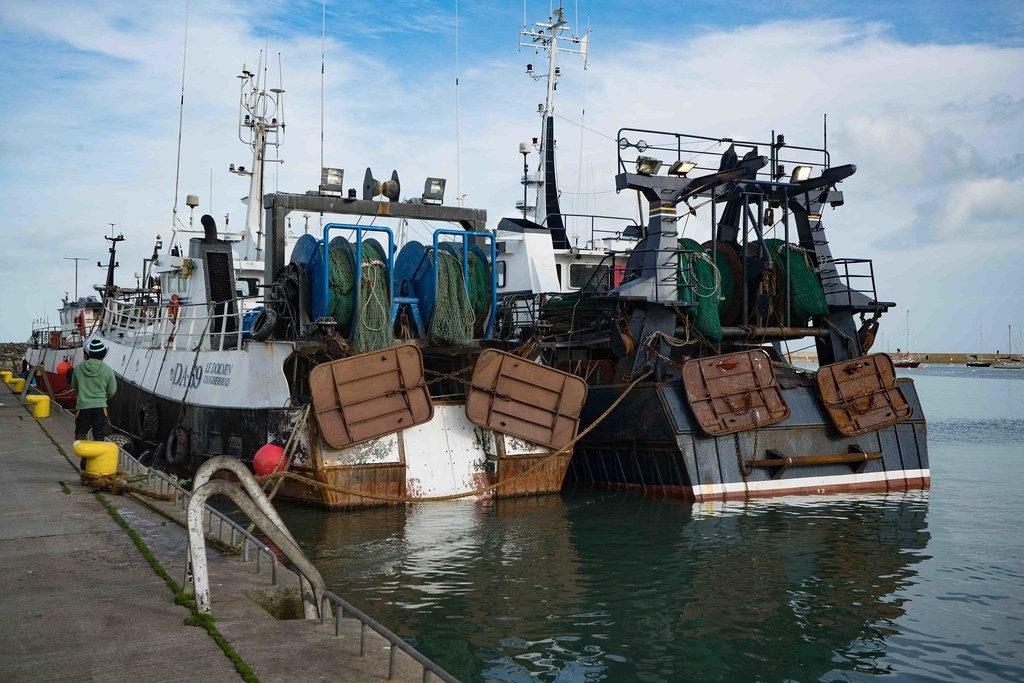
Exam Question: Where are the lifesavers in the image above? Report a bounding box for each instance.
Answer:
[167,293,180,324]
[136,402,159,441]
[249,307,278,342]
[165,425,188,466]
[16,362,23,373]
[73,315,80,326]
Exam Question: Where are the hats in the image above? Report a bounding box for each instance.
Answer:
[89,340,107,359]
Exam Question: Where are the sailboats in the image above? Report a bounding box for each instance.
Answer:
[992,324,1024,369]
[965,326,992,367]
[894,310,921,368]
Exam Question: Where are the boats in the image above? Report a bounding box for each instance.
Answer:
[19,1,935,515]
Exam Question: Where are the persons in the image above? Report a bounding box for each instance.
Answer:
[71,339,117,471]
